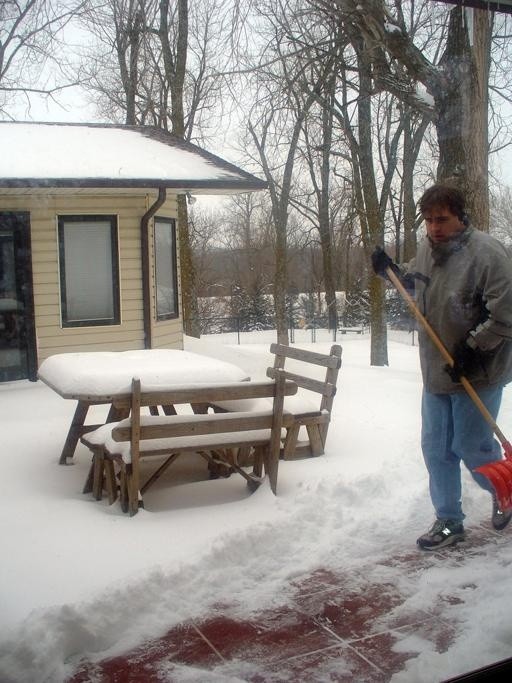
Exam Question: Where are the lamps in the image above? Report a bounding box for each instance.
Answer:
[185,192,197,204]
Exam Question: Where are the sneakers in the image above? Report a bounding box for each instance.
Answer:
[491,490,512,530]
[417,516,466,551]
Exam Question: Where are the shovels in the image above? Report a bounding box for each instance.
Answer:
[384,264,512,513]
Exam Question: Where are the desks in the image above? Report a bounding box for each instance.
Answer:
[36,349,251,465]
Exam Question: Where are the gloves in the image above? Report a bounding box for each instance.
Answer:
[442,341,478,384]
[371,244,399,281]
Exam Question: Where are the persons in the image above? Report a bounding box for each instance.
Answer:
[367,182,511,549]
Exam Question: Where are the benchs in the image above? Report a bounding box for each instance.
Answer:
[338,328,362,334]
[209,341,343,463]
[83,368,292,515]
